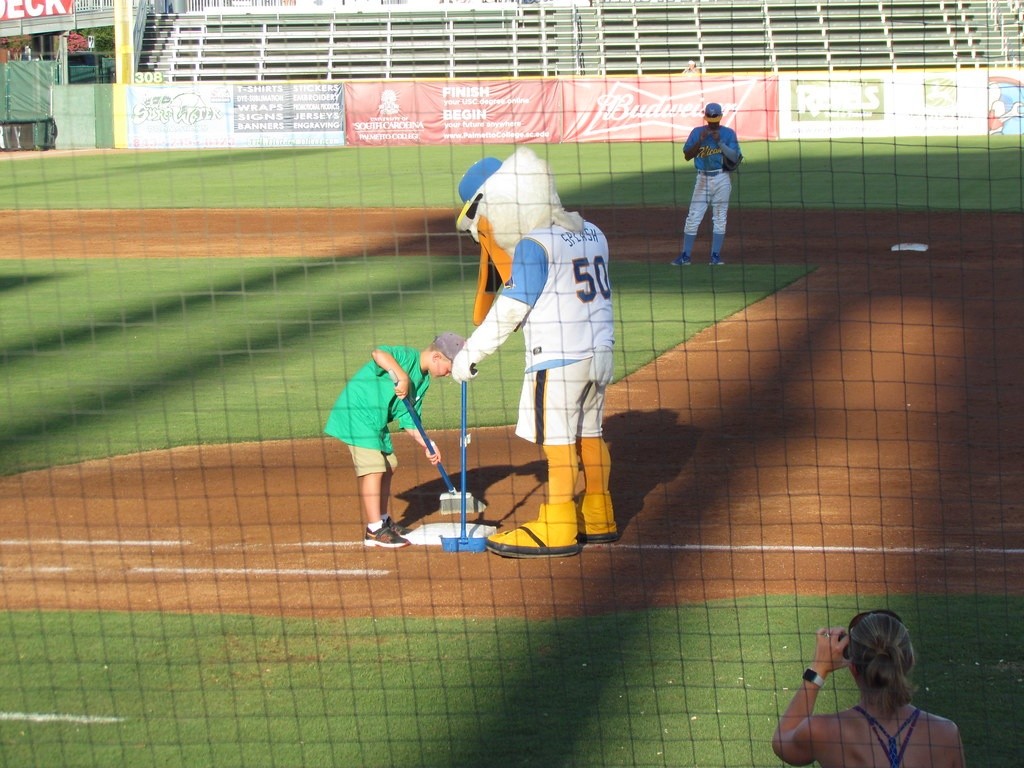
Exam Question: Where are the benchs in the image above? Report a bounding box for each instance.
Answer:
[134,0,1024,81]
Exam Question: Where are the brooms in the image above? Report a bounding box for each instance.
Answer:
[388,367,487,516]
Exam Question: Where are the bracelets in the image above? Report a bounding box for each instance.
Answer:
[423,441,436,448]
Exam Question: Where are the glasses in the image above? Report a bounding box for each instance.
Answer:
[705,113,719,118]
[847,609,907,652]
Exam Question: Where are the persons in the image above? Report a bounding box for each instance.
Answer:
[322,330,466,551]
[772,607,967,768]
[670,103,744,267]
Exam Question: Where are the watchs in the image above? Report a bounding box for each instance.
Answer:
[801,668,825,688]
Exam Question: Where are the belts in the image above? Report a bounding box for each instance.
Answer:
[698,171,719,176]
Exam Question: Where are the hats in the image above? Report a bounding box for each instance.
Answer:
[434,332,467,360]
[703,103,723,122]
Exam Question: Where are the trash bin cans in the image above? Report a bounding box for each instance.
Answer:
[0,117,57,151]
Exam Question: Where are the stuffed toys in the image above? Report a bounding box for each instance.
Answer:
[450,145,621,560]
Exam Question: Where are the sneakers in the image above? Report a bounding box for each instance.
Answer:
[364,526,411,548]
[709,253,724,265]
[382,515,406,535]
[671,249,691,265]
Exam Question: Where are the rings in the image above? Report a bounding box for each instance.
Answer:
[824,633,830,637]
